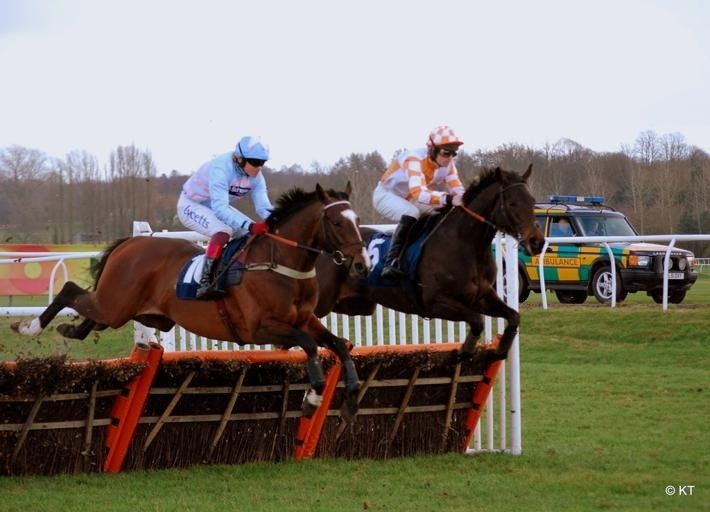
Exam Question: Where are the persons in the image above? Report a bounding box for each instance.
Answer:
[176,135,275,298]
[372,124,468,284]
[557,220,574,236]
[588,219,600,236]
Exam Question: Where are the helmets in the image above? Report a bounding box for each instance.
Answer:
[427,125,464,146]
[235,137,269,160]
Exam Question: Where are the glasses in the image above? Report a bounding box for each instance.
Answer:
[430,136,457,158]
[239,142,264,166]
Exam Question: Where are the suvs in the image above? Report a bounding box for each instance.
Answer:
[486,192,696,304]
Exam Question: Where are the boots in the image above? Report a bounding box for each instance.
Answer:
[196,256,227,300]
[381,222,411,275]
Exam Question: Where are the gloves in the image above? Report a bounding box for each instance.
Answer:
[452,195,464,206]
[251,223,268,235]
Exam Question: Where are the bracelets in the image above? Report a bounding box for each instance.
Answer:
[249,222,253,231]
[446,193,454,205]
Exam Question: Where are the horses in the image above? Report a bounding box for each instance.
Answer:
[314,163,546,366]
[9,180,373,423]
